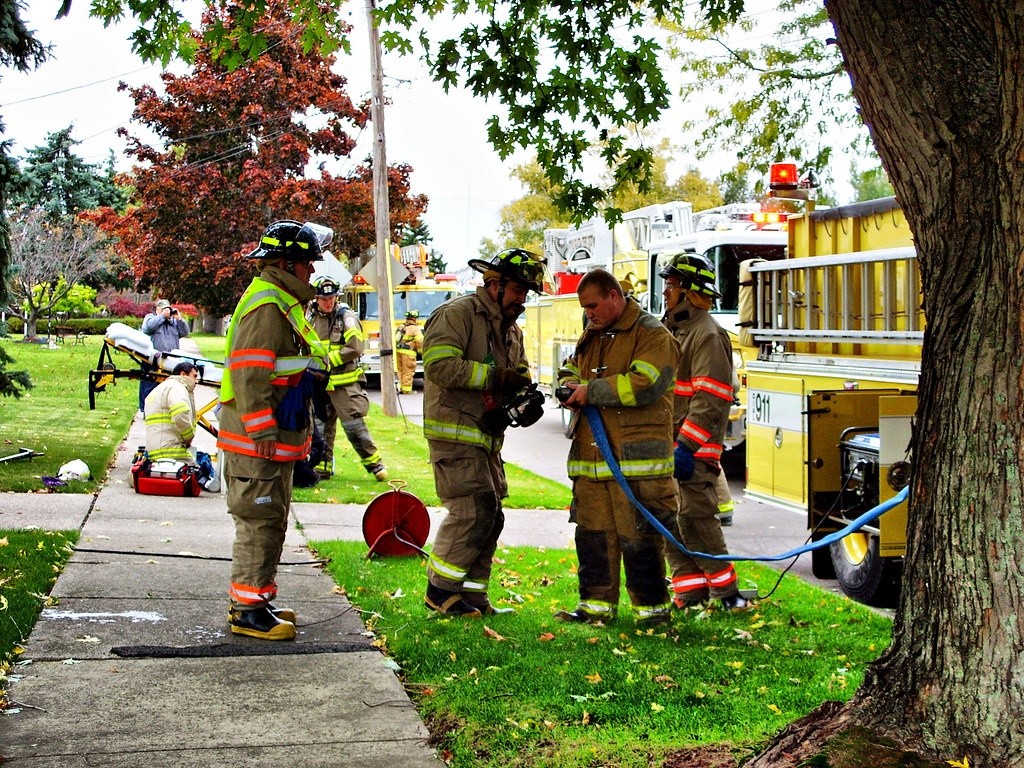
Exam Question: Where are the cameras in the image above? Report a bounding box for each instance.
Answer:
[162,307,177,316]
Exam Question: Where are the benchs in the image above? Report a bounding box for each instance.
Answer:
[54,326,87,346]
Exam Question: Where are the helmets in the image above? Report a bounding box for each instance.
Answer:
[313,275,340,297]
[468,248,551,295]
[248,220,324,261]
[660,252,725,300]
[406,309,419,318]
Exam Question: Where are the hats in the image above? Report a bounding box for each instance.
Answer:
[156,299,171,309]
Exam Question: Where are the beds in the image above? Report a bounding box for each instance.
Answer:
[88,322,226,437]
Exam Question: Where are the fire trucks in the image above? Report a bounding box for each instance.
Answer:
[346,242,467,390]
[518,199,789,480]
[735,194,927,607]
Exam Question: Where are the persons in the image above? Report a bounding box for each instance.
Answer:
[306,275,389,482]
[217,220,334,641]
[421,248,547,618]
[144,362,198,464]
[139,300,189,412]
[714,365,741,526]
[555,269,684,634]
[658,253,750,617]
[394,309,424,395]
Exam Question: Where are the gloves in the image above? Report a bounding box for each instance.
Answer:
[674,442,694,482]
[497,365,530,390]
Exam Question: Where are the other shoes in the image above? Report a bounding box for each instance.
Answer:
[403,390,416,394]
[556,607,612,626]
[426,584,498,617]
[229,603,296,639]
[721,588,747,612]
[375,468,388,480]
[720,516,732,526]
[642,616,677,635]
[671,592,708,612]
